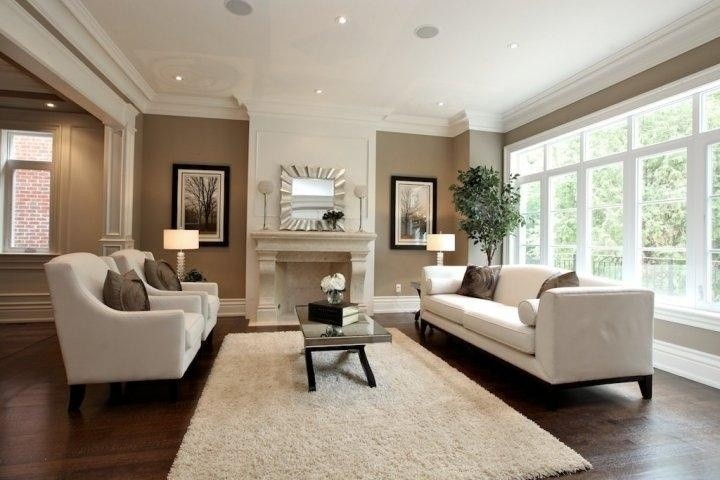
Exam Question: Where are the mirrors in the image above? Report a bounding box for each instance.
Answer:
[278,162,347,233]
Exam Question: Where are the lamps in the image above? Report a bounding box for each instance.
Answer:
[353,184,369,235]
[258,180,275,231]
[426,230,455,266]
[163,225,200,281]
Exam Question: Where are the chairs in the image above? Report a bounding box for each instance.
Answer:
[42,251,203,411]
[108,247,219,343]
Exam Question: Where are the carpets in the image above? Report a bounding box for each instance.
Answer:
[164,327,592,480]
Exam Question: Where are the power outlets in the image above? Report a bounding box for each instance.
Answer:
[395,284,402,293]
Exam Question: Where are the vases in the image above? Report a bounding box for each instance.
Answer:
[327,290,344,304]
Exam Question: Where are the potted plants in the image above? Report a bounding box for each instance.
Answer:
[321,210,344,231]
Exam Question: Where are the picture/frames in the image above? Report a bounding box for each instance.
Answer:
[170,163,230,247]
[389,175,438,250]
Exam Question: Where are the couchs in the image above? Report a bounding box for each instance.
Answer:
[419,263,655,411]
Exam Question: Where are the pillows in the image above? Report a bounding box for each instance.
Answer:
[145,257,183,291]
[517,299,539,327]
[103,268,150,311]
[536,271,579,299]
[454,264,501,301]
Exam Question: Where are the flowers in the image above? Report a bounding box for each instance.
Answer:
[319,272,346,303]
